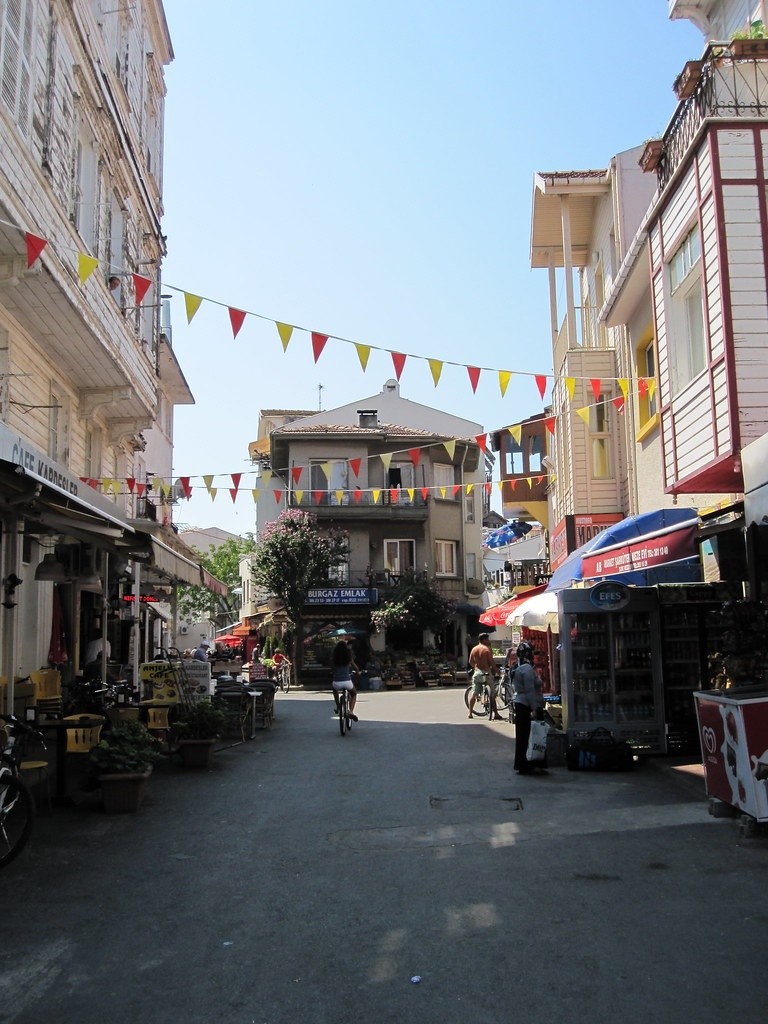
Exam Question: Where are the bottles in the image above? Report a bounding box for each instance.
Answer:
[573,614,652,720]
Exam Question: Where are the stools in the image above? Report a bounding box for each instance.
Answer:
[19,760,53,817]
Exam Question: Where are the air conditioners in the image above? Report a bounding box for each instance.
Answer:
[161,487,176,503]
[180,626,189,635]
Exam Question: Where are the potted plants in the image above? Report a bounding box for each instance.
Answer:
[673,60,703,100]
[636,138,663,174]
[88,721,168,815]
[180,698,226,767]
[727,19,768,58]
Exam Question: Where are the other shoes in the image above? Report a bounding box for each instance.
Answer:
[333,708,339,714]
[469,715,473,718]
[348,714,358,722]
[519,767,549,776]
[494,715,502,719]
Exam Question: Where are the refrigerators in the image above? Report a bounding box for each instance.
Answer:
[652,582,739,756]
[557,586,665,756]
[693,682,768,819]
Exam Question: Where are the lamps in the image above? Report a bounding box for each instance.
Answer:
[17,530,65,583]
[1,574,23,609]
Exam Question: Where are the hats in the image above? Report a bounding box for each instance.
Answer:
[256,643,260,646]
[201,641,211,648]
[275,648,281,653]
[517,643,535,661]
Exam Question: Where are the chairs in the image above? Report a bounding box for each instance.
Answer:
[29,669,277,754]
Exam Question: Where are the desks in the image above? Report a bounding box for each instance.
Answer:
[28,718,107,809]
[116,701,177,723]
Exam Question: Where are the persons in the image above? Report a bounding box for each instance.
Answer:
[211,642,234,662]
[225,643,233,652]
[183,649,191,655]
[252,644,260,663]
[330,641,359,722]
[194,641,211,662]
[85,632,111,666]
[511,642,543,775]
[467,633,502,719]
[235,645,240,656]
[272,648,290,674]
[191,646,197,654]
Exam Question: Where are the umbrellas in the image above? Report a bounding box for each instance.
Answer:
[506,591,560,634]
[214,635,241,641]
[478,598,531,627]
[482,521,532,558]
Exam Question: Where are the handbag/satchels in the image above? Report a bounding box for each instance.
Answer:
[526,720,551,760]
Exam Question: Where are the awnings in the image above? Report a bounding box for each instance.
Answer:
[544,508,701,593]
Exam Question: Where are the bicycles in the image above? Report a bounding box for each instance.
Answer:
[0,713,49,860]
[332,670,363,736]
[464,665,515,720]
[270,664,294,694]
